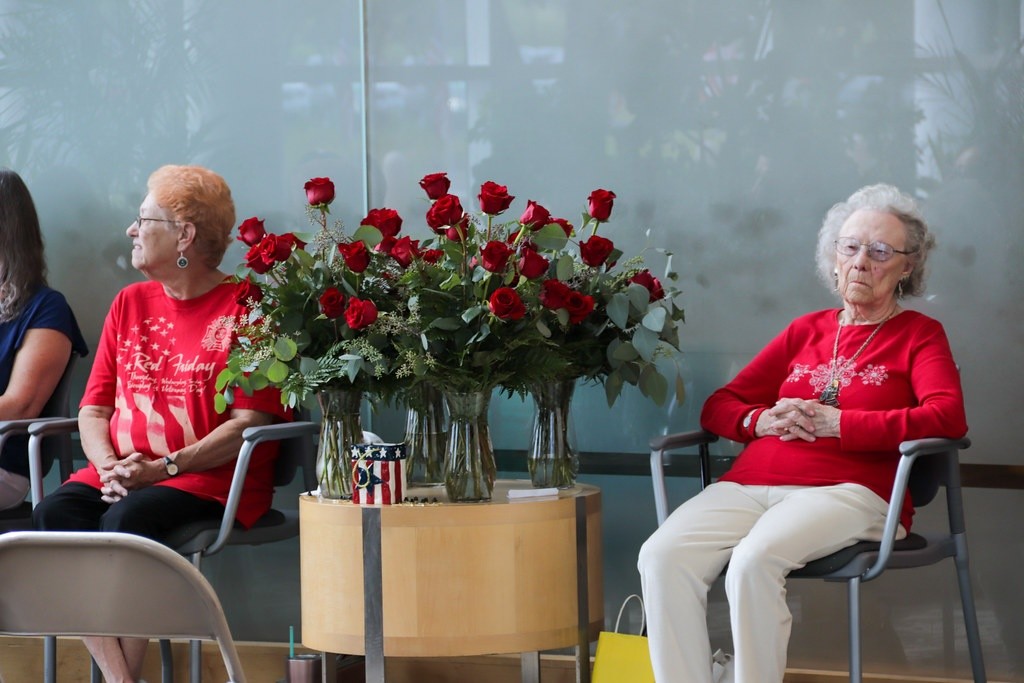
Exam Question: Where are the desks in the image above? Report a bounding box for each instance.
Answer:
[297,480,605,683]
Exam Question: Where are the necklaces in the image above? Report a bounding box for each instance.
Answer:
[820,304,900,408]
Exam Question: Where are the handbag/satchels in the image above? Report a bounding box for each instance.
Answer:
[590,595,656,683]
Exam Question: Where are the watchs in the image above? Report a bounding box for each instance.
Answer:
[162,456,179,477]
[742,409,758,431]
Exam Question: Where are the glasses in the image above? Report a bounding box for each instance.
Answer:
[833,237,911,262]
[135,214,180,231]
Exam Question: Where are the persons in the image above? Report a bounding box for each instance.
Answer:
[31,163,297,683]
[0,172,89,512]
[635,181,969,683]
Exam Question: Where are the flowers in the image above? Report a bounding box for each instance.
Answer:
[213,172,689,501]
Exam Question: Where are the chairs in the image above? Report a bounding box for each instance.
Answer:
[651,365,987,683]
[0,348,322,683]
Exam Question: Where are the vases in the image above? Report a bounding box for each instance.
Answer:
[403,385,448,489]
[316,389,366,504]
[442,390,497,505]
[526,379,580,490]
